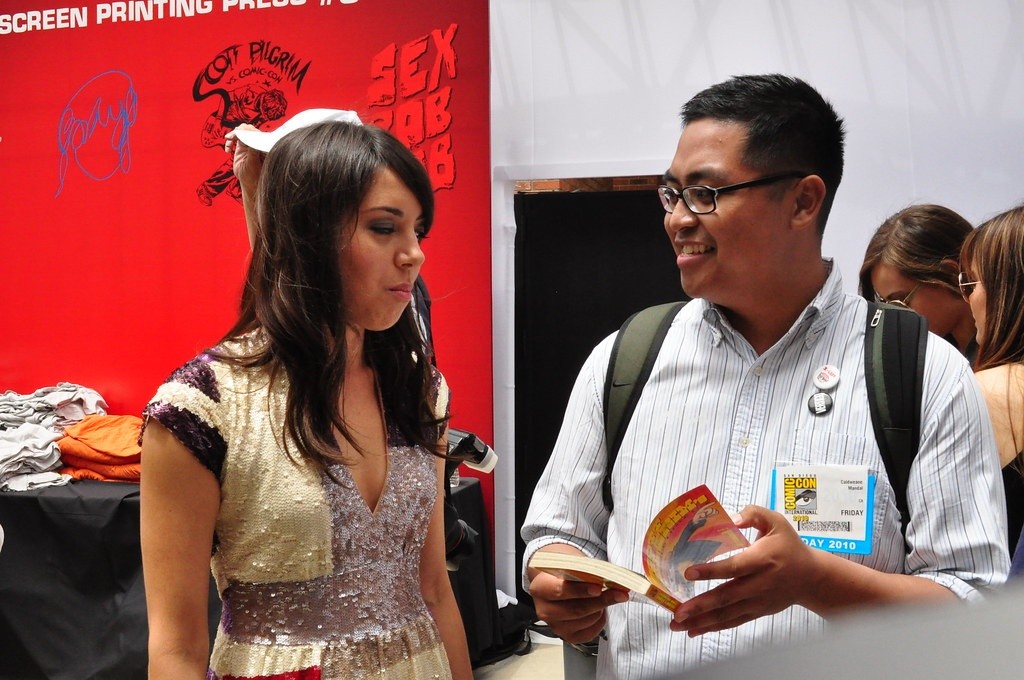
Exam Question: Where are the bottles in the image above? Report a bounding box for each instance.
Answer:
[449,469,459,488]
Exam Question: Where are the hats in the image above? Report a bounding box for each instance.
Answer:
[233,108,364,154]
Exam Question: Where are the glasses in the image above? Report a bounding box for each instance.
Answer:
[656,171,835,215]
[958,272,983,304]
[879,283,922,310]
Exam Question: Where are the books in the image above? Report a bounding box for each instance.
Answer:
[529,484,751,615]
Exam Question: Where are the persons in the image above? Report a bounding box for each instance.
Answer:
[857,204,1024,580]
[137,108,473,680]
[521,72,1011,680]
